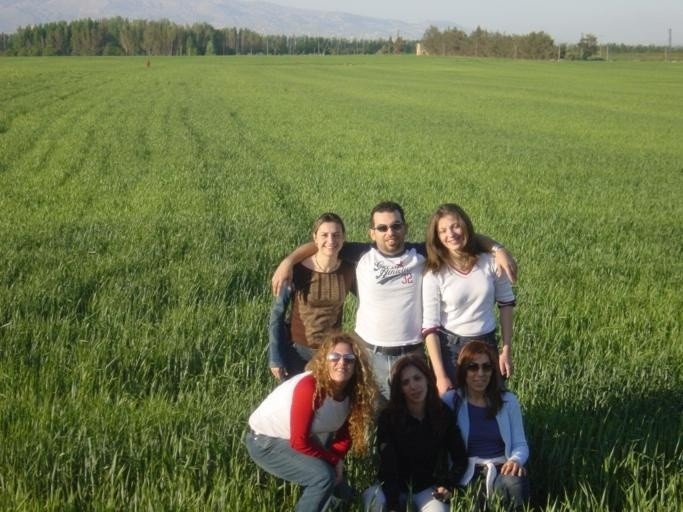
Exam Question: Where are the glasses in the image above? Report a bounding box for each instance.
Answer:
[465,362,494,372]
[326,352,358,364]
[372,222,405,232]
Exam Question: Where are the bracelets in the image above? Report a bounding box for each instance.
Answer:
[492,243,504,256]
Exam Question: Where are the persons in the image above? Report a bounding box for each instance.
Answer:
[268,211,358,384]
[361,355,468,512]
[441,340,530,509]
[423,203,516,396]
[241,335,377,512]
[272,202,518,452]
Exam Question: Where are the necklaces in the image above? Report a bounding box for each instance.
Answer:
[313,256,340,273]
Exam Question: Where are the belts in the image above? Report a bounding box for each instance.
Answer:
[356,334,424,356]
[246,426,263,438]
